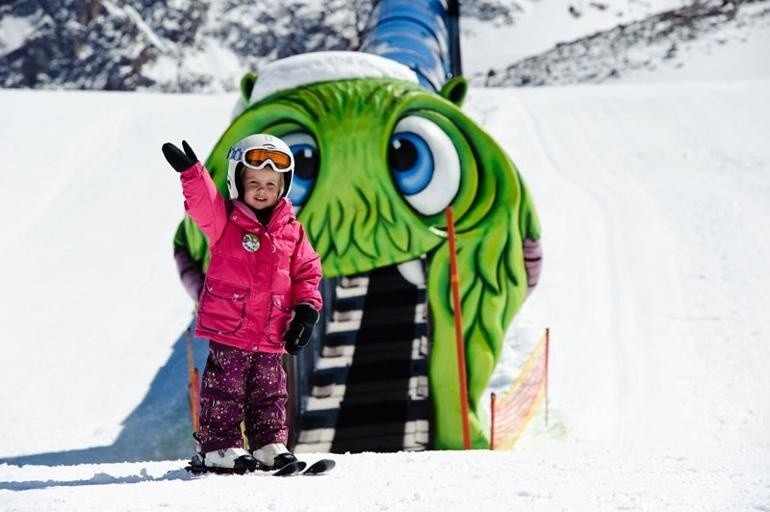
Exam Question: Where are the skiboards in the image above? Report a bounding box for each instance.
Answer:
[184,459,336,474]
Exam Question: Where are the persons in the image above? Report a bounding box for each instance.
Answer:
[161,133,323,469]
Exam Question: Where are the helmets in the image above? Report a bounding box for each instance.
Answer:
[226,132,295,201]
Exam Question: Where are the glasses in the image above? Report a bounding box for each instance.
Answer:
[226,146,295,173]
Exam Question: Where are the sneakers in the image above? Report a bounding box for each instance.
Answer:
[252,441,297,469]
[202,443,260,472]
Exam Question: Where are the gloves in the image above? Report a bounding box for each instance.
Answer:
[162,139,200,175]
[281,301,321,357]
